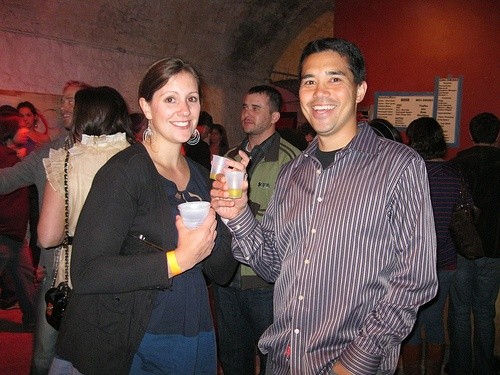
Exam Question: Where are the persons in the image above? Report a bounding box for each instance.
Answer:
[402,117,465,375]
[37,86,133,290]
[182,111,211,171]
[209,124,229,171]
[0,80,93,375]
[7,101,51,160]
[444,111,500,375]
[129,113,148,143]
[0,105,36,333]
[53,57,250,375]
[301,123,317,145]
[209,38,439,375]
[212,85,302,375]
[368,118,403,143]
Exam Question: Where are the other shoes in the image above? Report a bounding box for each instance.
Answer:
[23,321,35,331]
[0,295,18,308]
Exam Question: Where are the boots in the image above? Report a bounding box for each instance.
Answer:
[424,342,445,375]
[402,343,422,375]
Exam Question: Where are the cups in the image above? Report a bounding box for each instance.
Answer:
[178,201,210,229]
[210,154,230,180]
[225,171,244,199]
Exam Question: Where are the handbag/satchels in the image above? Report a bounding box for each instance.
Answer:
[45,282,70,332]
[448,162,485,259]
